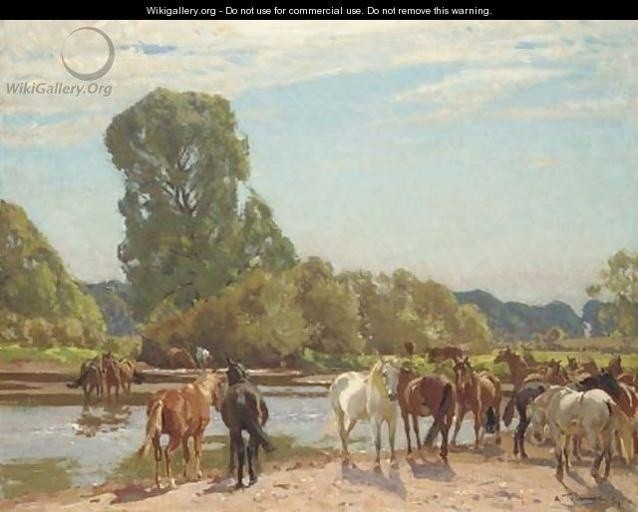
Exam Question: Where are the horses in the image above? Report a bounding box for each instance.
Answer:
[136,368,229,492]
[328,340,638,481]
[220,356,276,490]
[101,349,143,400]
[195,344,211,373]
[66,353,104,407]
[166,347,196,374]
